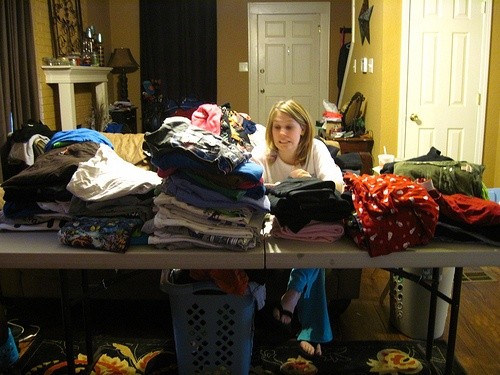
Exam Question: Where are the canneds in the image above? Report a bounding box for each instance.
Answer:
[87,27,92,38]
[91,51,99,66]
[90,24,95,34]
[98,33,102,42]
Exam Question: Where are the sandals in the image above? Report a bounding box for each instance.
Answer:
[299,338,324,362]
[272,294,295,326]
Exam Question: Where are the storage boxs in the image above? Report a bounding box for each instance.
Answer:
[159,268,256,375]
[420,178,440,199]
[326,121,342,133]
[325,269,362,306]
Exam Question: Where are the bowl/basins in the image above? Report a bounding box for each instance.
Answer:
[57,57,69,65]
[68,58,81,66]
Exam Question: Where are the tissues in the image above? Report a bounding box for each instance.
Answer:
[378,145,394,166]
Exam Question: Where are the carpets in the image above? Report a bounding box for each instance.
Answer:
[14,338,468,375]
[0,295,342,343]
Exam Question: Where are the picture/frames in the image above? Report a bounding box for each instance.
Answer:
[47,0,84,59]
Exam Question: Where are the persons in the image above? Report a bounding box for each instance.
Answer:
[252,101,343,358]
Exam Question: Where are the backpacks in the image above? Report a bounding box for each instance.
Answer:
[342,92,366,139]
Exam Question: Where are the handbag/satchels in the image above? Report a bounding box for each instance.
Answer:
[379,147,454,177]
[392,159,489,202]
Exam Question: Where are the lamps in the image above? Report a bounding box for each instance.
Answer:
[106,48,139,109]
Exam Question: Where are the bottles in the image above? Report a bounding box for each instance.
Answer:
[83,24,104,67]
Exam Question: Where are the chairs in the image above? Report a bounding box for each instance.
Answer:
[359,152,375,176]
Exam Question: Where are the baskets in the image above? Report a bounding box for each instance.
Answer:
[158,268,255,375]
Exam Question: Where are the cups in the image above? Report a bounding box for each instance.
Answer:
[90,51,100,66]
[378,154,394,166]
[83,52,90,65]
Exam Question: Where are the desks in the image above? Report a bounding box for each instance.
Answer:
[317,128,375,154]
[168,108,197,120]
[40,65,114,133]
[108,107,138,133]
[0,229,500,375]
